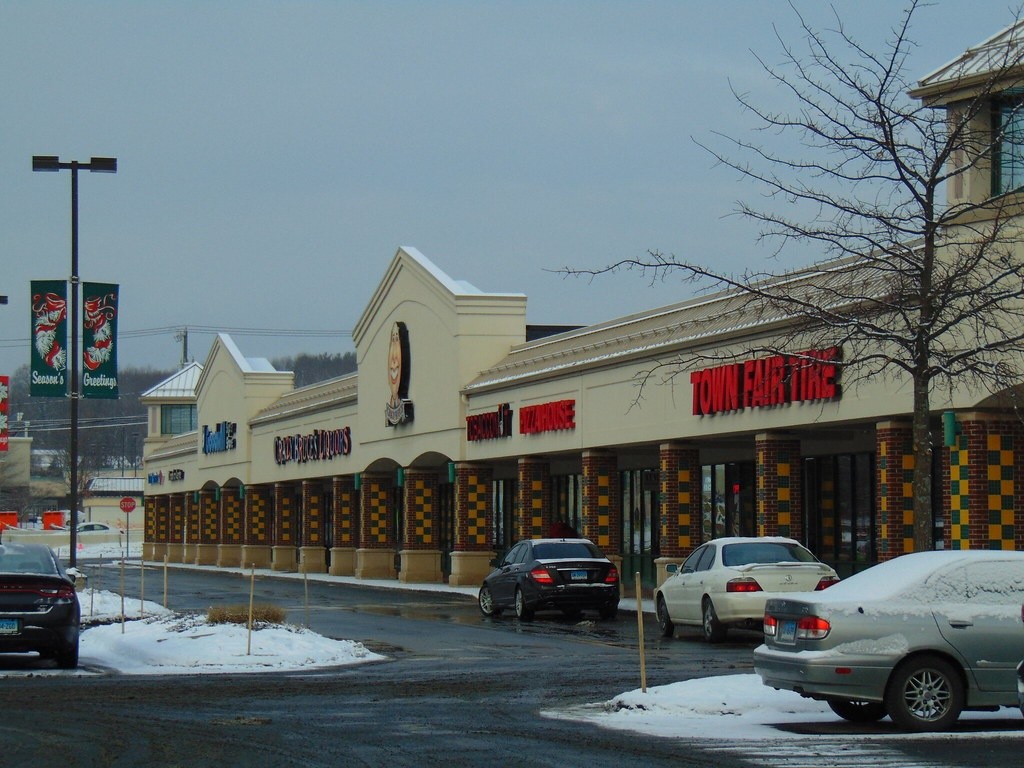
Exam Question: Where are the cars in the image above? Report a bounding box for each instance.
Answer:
[1,542,84,670]
[751,547,1024,732]
[76,522,127,535]
[655,536,839,643]
[479,537,623,625]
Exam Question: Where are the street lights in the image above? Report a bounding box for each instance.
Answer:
[31,152,120,573]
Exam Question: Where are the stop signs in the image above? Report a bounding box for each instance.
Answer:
[119,497,137,513]
